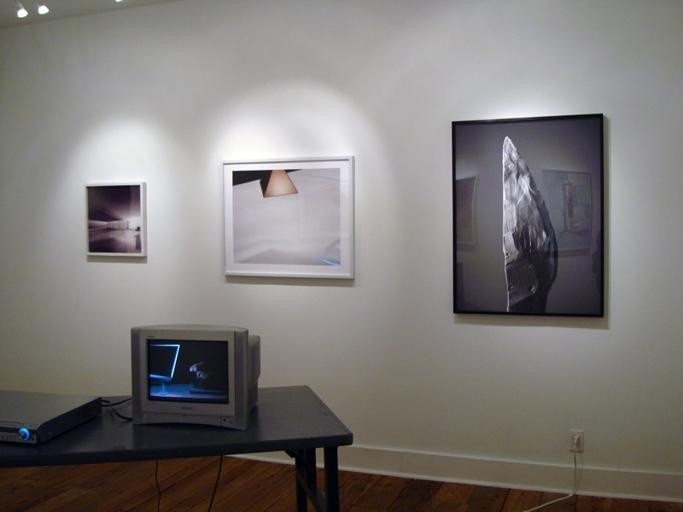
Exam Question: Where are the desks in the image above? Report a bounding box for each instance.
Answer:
[2,384,354,512]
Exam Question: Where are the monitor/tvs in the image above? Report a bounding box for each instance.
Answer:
[130,326,260,430]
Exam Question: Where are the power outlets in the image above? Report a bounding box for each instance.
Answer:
[567,429,584,452]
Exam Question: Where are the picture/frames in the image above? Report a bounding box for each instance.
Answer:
[452,112,604,319]
[223,157,355,280]
[87,183,147,258]
